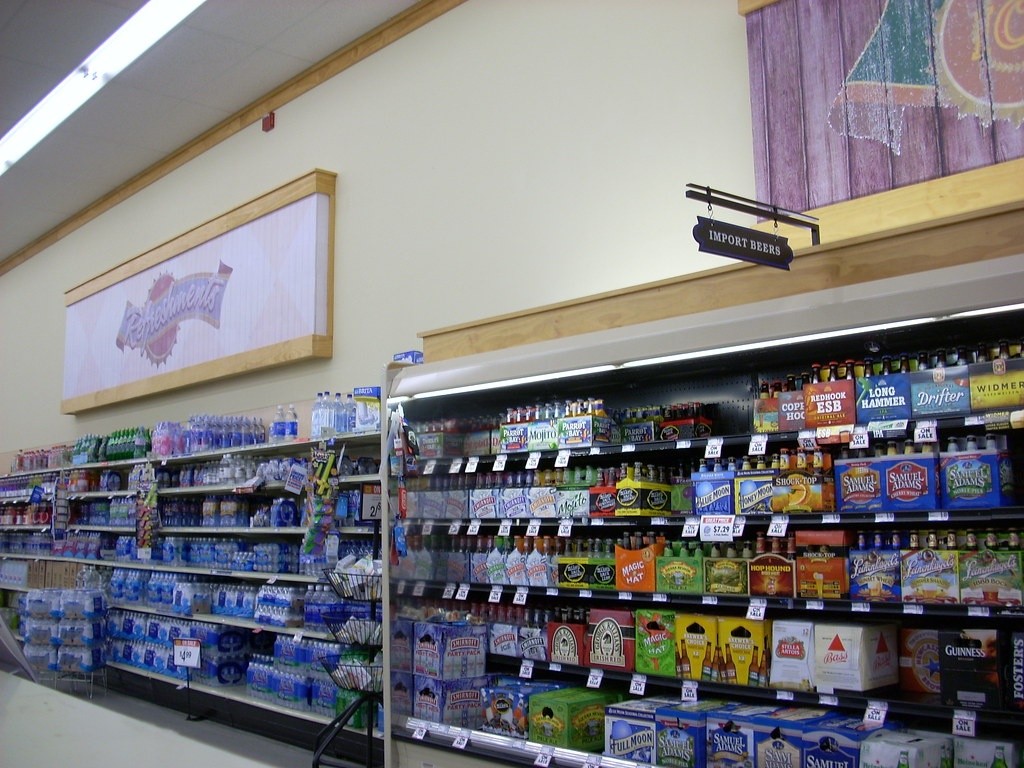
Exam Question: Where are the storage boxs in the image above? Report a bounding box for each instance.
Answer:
[392,355,1024,768]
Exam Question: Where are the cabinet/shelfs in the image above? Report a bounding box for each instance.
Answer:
[384,256,1024,768]
[0,432,382,768]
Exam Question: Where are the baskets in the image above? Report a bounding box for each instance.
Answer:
[321,567,383,603]
[319,653,384,694]
[320,611,383,650]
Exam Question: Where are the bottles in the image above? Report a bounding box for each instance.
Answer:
[725,643,738,685]
[712,647,719,682]
[719,648,726,683]
[747,646,758,686]
[681,639,692,679]
[897,749,909,767]
[2,339,1024,767]
[990,746,1008,767]
[947,747,953,767]
[940,744,947,767]
[674,642,682,677]
[759,650,767,687]
[701,641,711,682]
